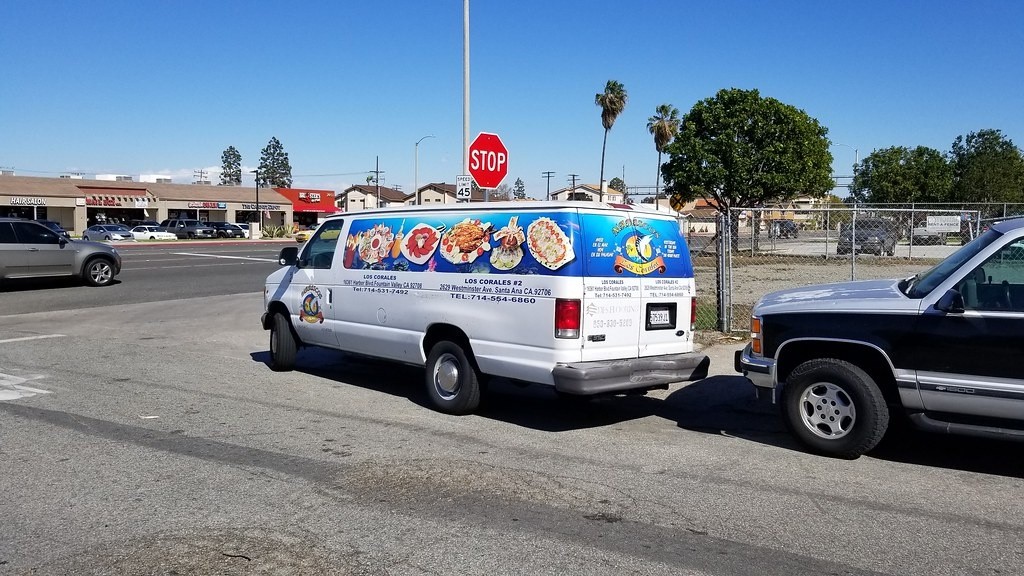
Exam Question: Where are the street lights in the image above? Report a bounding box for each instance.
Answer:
[832,143,858,177]
[250,170,262,232]
[414,133,438,207]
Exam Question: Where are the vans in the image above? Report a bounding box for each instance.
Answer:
[263,203,710,415]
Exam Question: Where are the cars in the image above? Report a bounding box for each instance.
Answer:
[33,219,70,241]
[961,217,1024,260]
[129,226,178,241]
[911,221,947,245]
[733,216,1023,461]
[81,225,134,242]
[768,220,801,239]
[234,223,263,239]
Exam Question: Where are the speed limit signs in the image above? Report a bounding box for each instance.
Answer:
[456,174,473,199]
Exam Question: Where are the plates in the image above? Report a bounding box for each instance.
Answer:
[490,247,522,270]
[400,223,441,265]
[441,221,490,263]
[359,228,393,264]
[527,218,575,270]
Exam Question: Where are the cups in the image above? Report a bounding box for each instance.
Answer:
[345,246,355,268]
[392,239,402,258]
[371,237,381,249]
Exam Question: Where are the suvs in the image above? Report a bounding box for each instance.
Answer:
[203,221,243,240]
[836,217,899,256]
[160,219,218,240]
[126,220,161,228]
[0,217,121,287]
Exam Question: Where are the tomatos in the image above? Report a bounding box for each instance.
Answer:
[530,221,566,263]
[443,220,491,261]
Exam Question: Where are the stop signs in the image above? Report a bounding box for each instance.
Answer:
[466,132,509,189]
